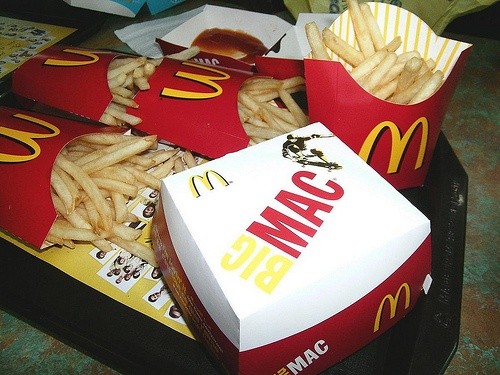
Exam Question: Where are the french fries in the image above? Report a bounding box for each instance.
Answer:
[44,131,199,268]
[238,75,308,147]
[99,45,200,126]
[304,0,444,104]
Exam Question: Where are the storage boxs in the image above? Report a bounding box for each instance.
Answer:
[148,122,432,375]
